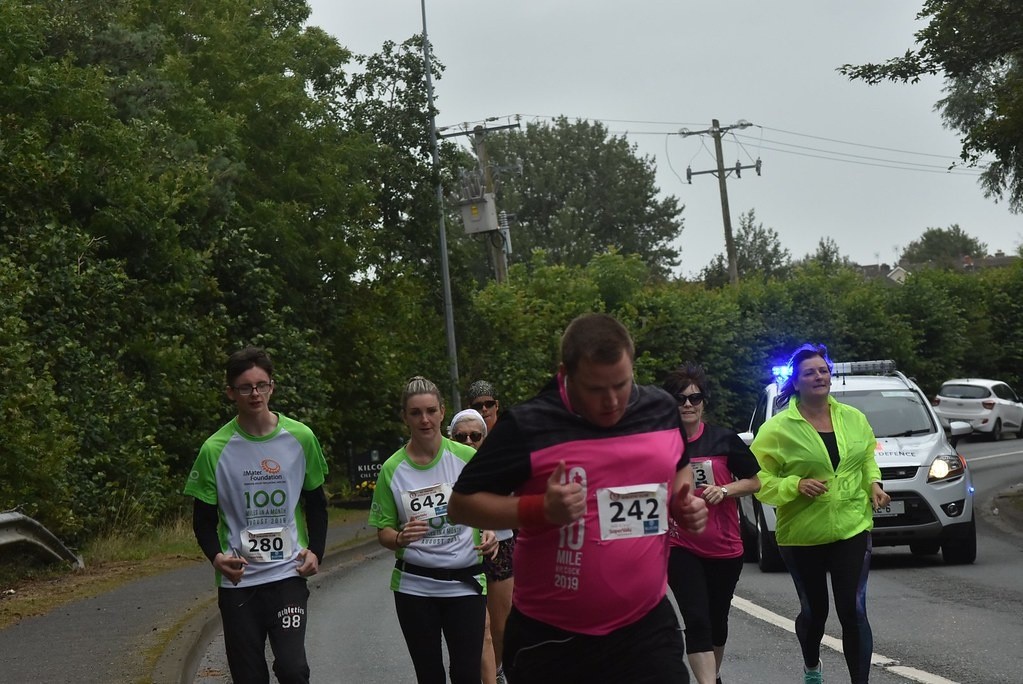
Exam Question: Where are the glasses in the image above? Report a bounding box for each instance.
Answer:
[453,432,484,444]
[672,393,706,407]
[230,379,272,396]
[469,400,496,411]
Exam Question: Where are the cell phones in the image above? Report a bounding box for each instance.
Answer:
[230,549,243,586]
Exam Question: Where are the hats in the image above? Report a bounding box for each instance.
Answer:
[467,380,496,405]
[451,409,487,437]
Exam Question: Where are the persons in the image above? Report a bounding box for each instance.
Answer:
[449,409,515,684]
[463,380,498,437]
[368,376,499,684]
[445,314,708,684]
[750,345,891,684]
[183,349,329,684]
[667,365,761,684]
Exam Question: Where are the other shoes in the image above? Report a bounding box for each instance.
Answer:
[804,658,824,684]
[716,676,722,684]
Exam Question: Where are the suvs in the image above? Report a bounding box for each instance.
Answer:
[737,360,977,573]
[932,378,1023,441]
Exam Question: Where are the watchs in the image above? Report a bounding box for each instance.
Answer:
[720,486,727,496]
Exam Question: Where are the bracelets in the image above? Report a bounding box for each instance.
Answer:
[396,532,401,546]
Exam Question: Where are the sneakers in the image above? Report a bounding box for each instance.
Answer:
[495,660,507,684]
